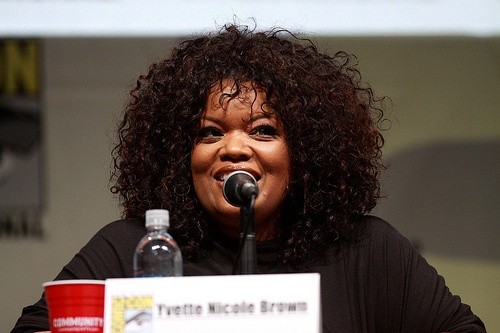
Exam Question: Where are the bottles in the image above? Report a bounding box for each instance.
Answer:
[132,209,182,276]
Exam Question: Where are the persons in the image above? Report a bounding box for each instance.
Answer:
[10,14,487,333]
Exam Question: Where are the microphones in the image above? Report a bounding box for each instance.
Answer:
[222,170,258,206]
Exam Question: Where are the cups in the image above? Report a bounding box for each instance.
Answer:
[42,280,106,333]
